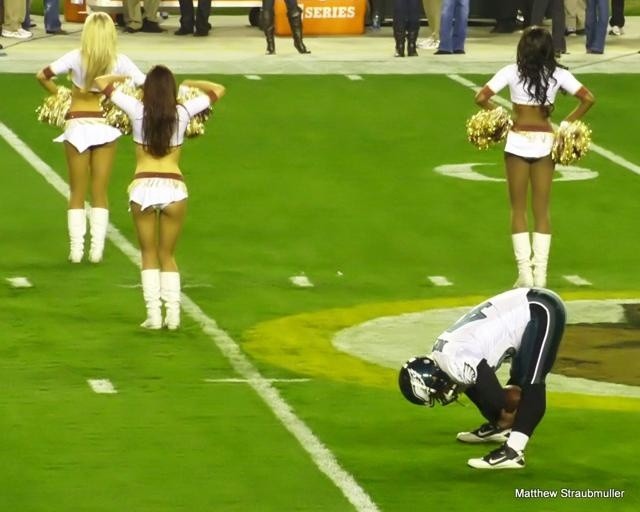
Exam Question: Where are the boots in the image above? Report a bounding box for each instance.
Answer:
[141,269,162,329]
[288,7,310,53]
[261,9,276,55]
[394,32,406,57]
[532,232,552,289]
[160,272,181,330]
[67,209,87,263]
[88,208,109,262]
[512,232,534,287]
[408,30,418,57]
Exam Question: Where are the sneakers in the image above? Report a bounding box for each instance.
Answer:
[609,26,624,36]
[467,441,526,469]
[2,22,65,37]
[456,422,512,443]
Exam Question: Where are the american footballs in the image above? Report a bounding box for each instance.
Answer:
[501,385,521,413]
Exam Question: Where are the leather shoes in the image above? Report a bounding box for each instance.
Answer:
[124,18,208,35]
[434,51,451,55]
[454,49,465,54]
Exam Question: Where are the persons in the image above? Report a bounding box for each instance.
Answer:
[399,287,566,473]
[121,0,167,34]
[393,0,421,56]
[34,11,146,265]
[490,0,626,59]
[173,0,212,36]
[0,0,67,51]
[473,26,595,291]
[433,0,471,54]
[262,0,311,55]
[94,64,227,333]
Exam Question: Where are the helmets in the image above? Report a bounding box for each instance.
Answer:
[400,356,459,407]
